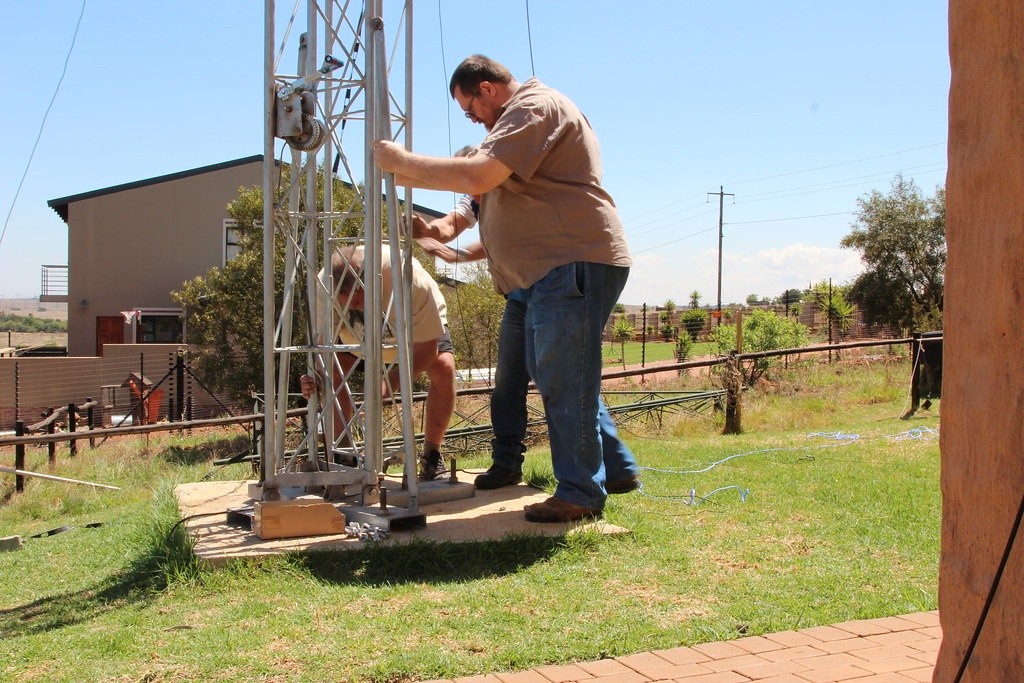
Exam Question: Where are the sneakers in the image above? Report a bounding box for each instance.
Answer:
[524,493,602,522]
[474,463,523,490]
[419,449,447,483]
[604,474,642,495]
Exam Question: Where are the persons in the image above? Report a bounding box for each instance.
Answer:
[375,57,648,522]
[404,145,540,492]
[301,240,455,482]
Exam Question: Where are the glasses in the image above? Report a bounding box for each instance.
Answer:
[465,90,477,121]
